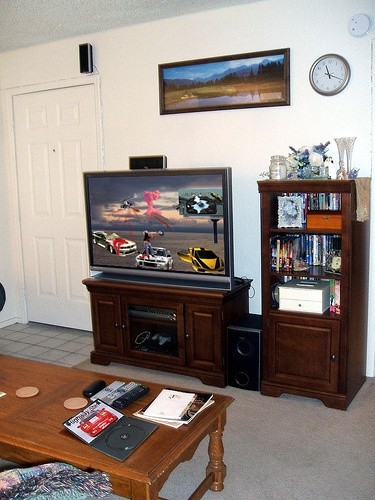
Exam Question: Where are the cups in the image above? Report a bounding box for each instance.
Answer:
[269,155,291,179]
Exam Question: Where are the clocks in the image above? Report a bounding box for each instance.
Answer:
[309,53,351,96]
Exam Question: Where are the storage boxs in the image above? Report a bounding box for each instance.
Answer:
[278,280,332,316]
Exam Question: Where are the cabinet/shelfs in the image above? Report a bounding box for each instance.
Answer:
[257,180,369,410]
[80,278,255,389]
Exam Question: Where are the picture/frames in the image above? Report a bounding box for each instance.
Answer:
[158,48,290,115]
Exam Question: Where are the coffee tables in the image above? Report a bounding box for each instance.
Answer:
[0,353,235,500]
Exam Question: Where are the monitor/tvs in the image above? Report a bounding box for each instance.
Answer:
[83,167,235,291]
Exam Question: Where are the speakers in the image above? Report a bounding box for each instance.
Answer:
[79,43,93,74]
[226,313,262,391]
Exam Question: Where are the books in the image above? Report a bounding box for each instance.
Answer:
[269,192,342,315]
[133,387,215,428]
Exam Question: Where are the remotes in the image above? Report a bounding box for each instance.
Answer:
[90,380,150,410]
